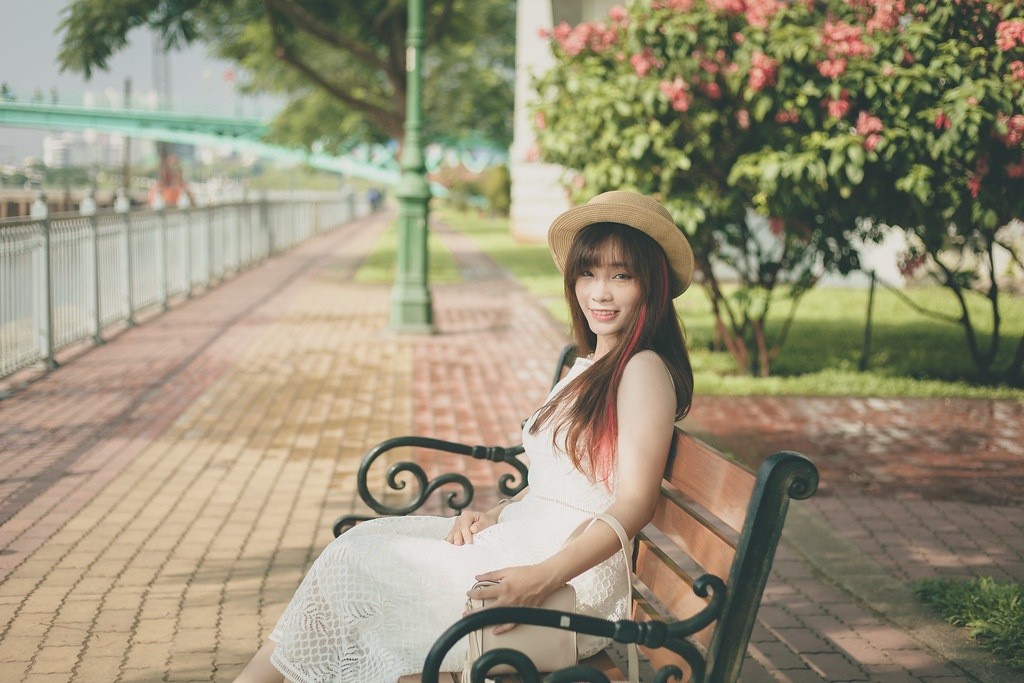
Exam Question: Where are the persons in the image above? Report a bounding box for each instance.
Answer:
[232,191,693,683]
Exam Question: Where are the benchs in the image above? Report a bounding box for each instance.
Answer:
[331,342,821,683]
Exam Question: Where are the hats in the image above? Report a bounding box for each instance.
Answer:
[547,190,695,300]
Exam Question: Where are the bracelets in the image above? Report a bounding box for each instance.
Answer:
[499,499,513,504]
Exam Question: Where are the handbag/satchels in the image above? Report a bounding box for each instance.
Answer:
[464,580,579,676]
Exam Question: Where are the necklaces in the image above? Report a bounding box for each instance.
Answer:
[587,353,594,359]
